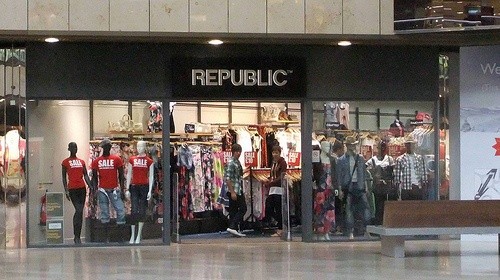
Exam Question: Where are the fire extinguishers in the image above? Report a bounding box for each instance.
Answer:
[40,190,48,226]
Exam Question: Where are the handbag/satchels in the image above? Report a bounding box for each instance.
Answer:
[342,188,349,204]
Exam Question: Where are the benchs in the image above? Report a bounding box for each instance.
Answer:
[366,200,500,258]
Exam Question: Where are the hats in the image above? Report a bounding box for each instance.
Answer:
[343,135,359,145]
[404,133,418,143]
[100,139,113,148]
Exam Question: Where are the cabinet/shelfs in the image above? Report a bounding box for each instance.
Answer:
[89,129,220,146]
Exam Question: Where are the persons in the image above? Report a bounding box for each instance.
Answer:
[61,142,91,242]
[223,145,250,238]
[314,140,338,240]
[365,139,395,226]
[339,137,369,240]
[124,141,154,244]
[393,135,430,201]
[265,148,286,236]
[325,101,351,128]
[91,140,127,228]
[390,118,404,138]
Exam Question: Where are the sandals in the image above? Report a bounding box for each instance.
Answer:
[270,232,282,237]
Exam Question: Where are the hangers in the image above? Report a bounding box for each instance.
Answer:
[415,121,434,132]
[345,128,376,139]
[243,166,302,183]
[229,122,300,133]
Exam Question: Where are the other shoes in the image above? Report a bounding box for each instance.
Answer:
[226,228,246,237]
